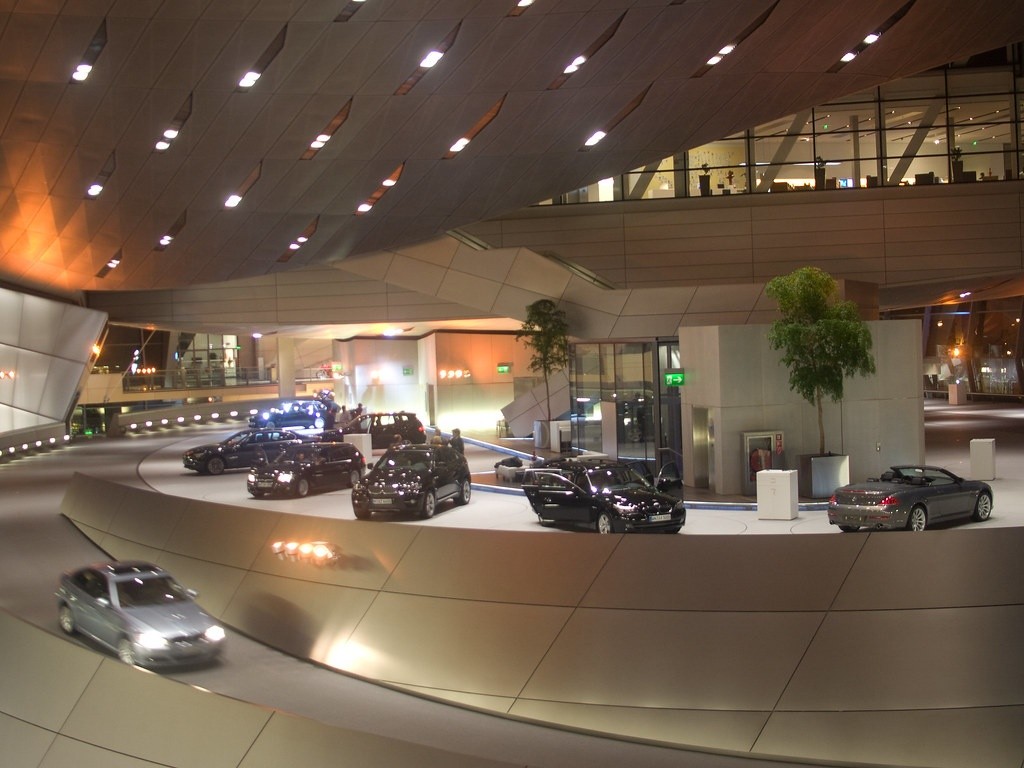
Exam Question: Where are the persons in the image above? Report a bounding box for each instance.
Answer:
[327,404,362,426]
[390,426,465,455]
[296,451,304,460]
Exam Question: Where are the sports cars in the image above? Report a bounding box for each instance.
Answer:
[826,465,995,532]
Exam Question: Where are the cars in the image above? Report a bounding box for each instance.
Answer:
[181,429,323,477]
[316,412,427,450]
[349,443,473,520]
[53,560,228,670]
[246,441,367,499]
[248,400,330,430]
[521,456,687,533]
